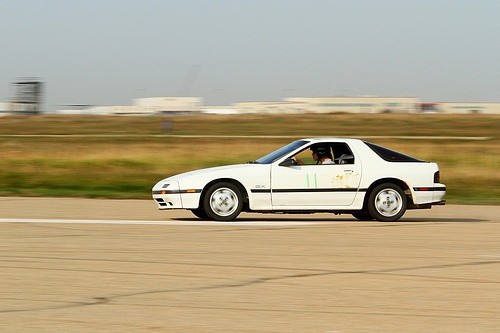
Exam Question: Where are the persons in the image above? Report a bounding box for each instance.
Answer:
[344,169,356,187]
[291,143,335,165]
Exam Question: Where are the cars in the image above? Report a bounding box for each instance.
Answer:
[152,136,447,222]
